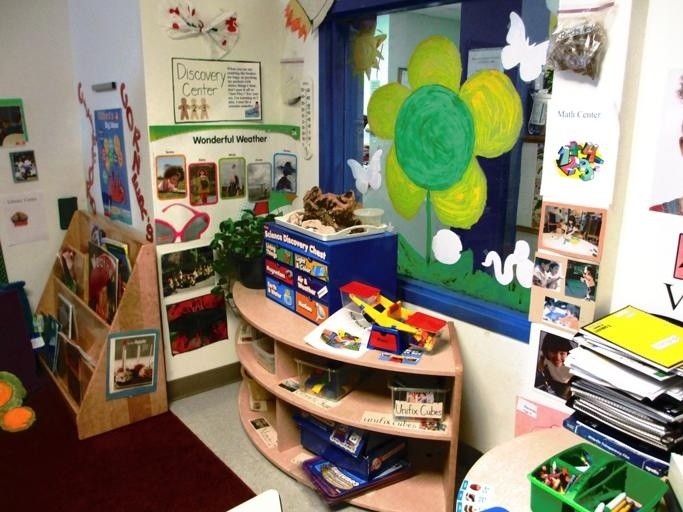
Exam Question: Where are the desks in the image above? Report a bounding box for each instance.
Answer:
[455,427,675,512]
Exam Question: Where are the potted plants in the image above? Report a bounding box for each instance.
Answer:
[209,209,283,299]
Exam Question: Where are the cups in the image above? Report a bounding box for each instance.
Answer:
[352,207,385,229]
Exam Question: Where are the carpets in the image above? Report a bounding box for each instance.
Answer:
[0,359,257,512]
[168,381,378,512]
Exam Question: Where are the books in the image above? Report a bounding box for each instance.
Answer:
[26,212,151,405]
[564,304,656,472]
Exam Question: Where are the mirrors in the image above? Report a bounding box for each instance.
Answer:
[317,1,562,344]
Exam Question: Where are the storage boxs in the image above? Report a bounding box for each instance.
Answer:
[525,443,669,512]
[240,365,276,412]
[292,357,359,403]
[287,408,423,482]
[251,337,275,374]
[385,381,447,423]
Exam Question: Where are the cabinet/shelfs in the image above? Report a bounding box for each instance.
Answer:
[261,221,398,326]
[231,280,463,512]
[33,210,167,440]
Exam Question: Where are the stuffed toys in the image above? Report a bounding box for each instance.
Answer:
[0,371,35,432]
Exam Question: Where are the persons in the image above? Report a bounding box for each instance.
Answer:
[545,262,562,289]
[543,299,565,321]
[16,153,31,179]
[538,332,578,401]
[194,169,212,204]
[533,259,552,288]
[560,215,579,241]
[228,164,242,194]
[159,165,186,195]
[574,266,597,301]
[557,304,578,329]
